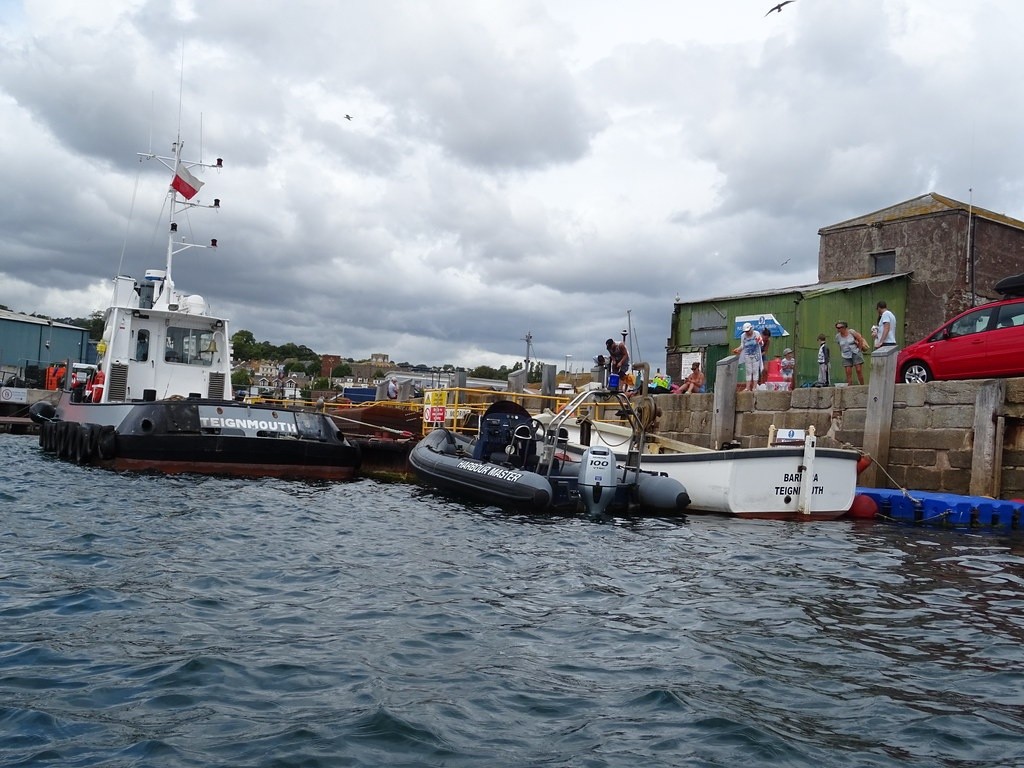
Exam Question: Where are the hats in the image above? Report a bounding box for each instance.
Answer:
[742,322,752,332]
[606,339,613,349]
[783,348,793,355]
[392,377,397,382]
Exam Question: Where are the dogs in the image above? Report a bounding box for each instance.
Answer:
[870,325,878,338]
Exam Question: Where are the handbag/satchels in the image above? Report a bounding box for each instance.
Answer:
[849,331,870,352]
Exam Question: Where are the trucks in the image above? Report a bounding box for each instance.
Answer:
[0,357,99,418]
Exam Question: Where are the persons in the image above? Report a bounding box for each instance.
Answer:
[734,322,764,392]
[387,377,398,399]
[618,371,637,401]
[816,333,832,387]
[835,322,864,385]
[871,301,897,352]
[779,348,795,391]
[606,339,629,386]
[734,327,771,385]
[670,362,705,395]
[165,351,180,362]
[136,333,148,361]
[593,354,611,388]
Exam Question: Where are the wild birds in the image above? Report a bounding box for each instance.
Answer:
[344,114,353,121]
[764,0,796,16]
[781,258,790,266]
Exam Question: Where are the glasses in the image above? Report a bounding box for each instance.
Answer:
[817,338,820,341]
[744,329,751,332]
[836,325,845,330]
[691,366,698,371]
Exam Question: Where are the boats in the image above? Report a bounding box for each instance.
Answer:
[29,37,353,482]
[531,408,862,522]
[408,373,692,517]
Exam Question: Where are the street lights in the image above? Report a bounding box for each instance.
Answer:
[565,355,572,378]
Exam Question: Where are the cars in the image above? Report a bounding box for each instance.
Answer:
[895,298,1024,384]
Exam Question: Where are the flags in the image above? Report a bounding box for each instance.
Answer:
[172,162,204,200]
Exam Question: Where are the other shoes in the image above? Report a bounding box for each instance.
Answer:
[685,391,691,394]
[752,388,756,391]
[743,389,750,391]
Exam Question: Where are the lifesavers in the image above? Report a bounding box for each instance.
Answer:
[78,429,88,465]
[92,371,105,404]
[70,374,79,386]
[38,421,78,464]
[86,423,98,454]
